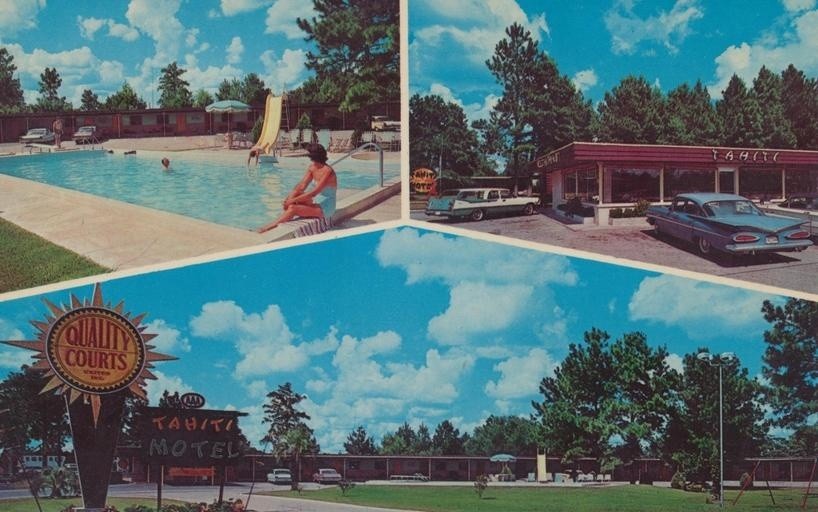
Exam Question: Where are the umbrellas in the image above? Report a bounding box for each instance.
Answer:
[491,454,518,473]
[205,99,254,131]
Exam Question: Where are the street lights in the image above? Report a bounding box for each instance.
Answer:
[696,350,736,503]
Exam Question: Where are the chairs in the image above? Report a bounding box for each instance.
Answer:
[213,127,401,157]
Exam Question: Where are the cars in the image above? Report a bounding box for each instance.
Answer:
[647,193,813,256]
[266,468,292,483]
[64,464,78,476]
[425,186,540,221]
[370,115,401,133]
[753,195,818,240]
[72,124,99,145]
[312,468,342,484]
[19,128,55,146]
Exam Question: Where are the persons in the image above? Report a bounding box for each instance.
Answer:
[246,141,269,166]
[52,114,65,147]
[257,143,338,232]
[161,156,171,171]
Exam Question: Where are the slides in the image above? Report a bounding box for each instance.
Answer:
[254,93,286,155]
[536,448,548,483]
